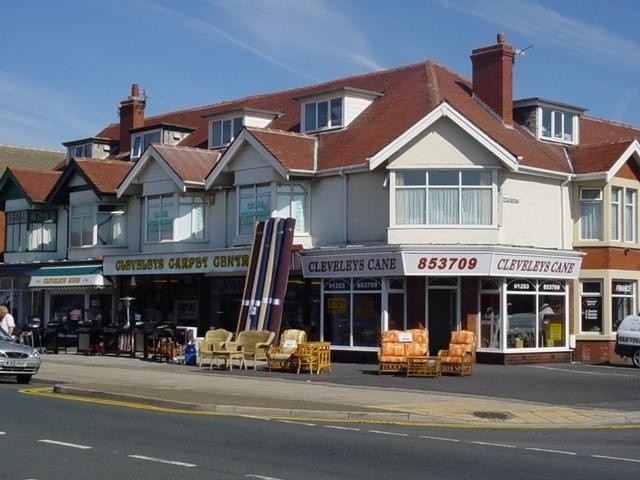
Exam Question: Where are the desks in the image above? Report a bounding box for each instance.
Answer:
[297,342,332,374]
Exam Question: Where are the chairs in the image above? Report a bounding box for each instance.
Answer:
[198,328,308,372]
[378,329,476,377]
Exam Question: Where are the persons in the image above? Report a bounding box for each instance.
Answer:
[0,305,16,338]
